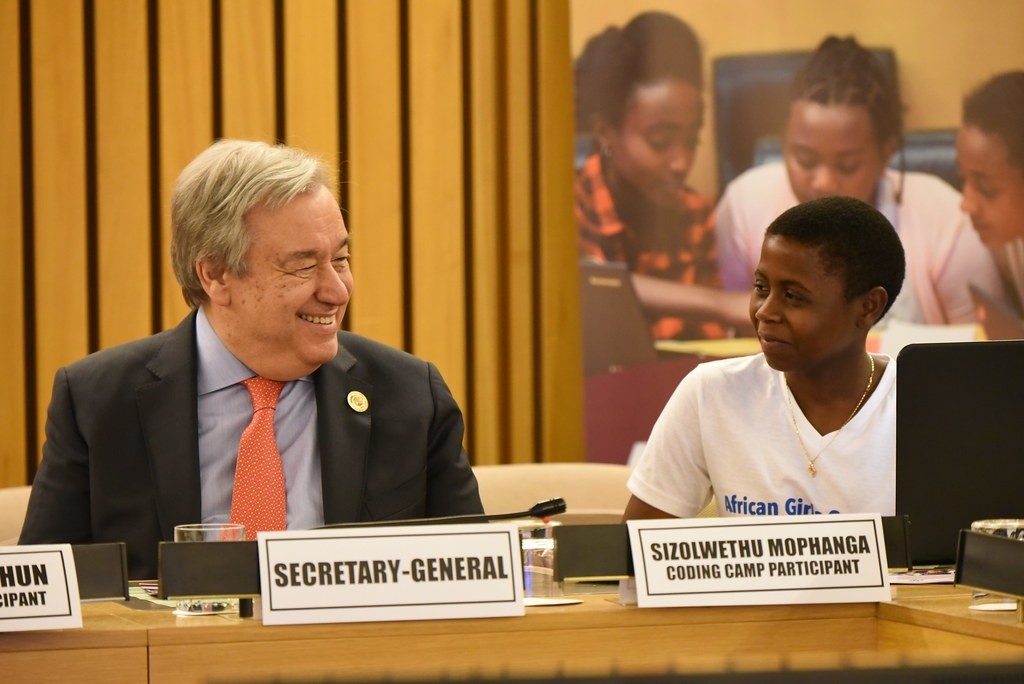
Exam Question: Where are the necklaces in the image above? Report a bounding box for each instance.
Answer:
[785,353,875,477]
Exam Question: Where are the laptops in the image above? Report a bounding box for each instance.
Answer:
[896,337,1024,564]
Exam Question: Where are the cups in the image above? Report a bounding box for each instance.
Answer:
[175,525,246,616]
[514,520,563,597]
[971,519,1024,541]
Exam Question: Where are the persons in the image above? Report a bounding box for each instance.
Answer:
[18,138,488,579]
[716,35,1004,326]
[956,70,1024,313]
[567,10,758,341]
[621,196,906,522]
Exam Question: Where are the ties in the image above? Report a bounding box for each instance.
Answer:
[220,375,287,542]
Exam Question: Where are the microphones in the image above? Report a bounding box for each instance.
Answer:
[309,498,567,530]
